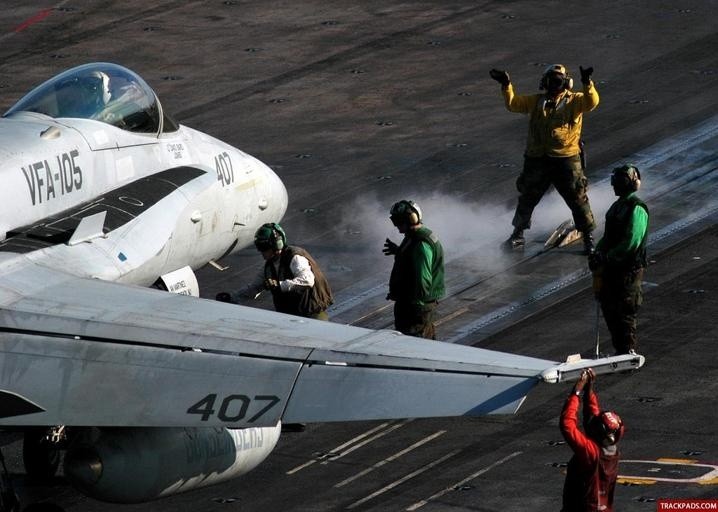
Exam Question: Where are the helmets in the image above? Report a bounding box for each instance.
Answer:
[587,409,624,447]
[611,163,641,196]
[390,200,423,226]
[256,222,288,253]
[542,64,568,92]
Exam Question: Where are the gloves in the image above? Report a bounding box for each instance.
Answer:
[412,308,426,325]
[263,278,280,290]
[579,65,593,86]
[489,68,511,86]
[216,292,231,302]
[383,238,399,255]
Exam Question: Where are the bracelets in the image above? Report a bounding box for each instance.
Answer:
[571,389,581,398]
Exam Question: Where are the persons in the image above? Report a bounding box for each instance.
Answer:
[215,222,334,322]
[558,368,624,512]
[381,199,445,341]
[586,163,650,365]
[488,63,599,255]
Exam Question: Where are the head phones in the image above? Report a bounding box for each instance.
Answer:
[612,167,641,192]
[264,223,284,256]
[400,199,423,226]
[597,410,615,447]
[538,66,574,90]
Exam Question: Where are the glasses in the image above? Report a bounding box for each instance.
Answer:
[254,239,271,252]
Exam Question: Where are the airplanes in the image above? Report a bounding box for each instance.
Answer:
[1,60,646,512]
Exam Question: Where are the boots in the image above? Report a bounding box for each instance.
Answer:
[501,227,525,253]
[582,232,596,255]
